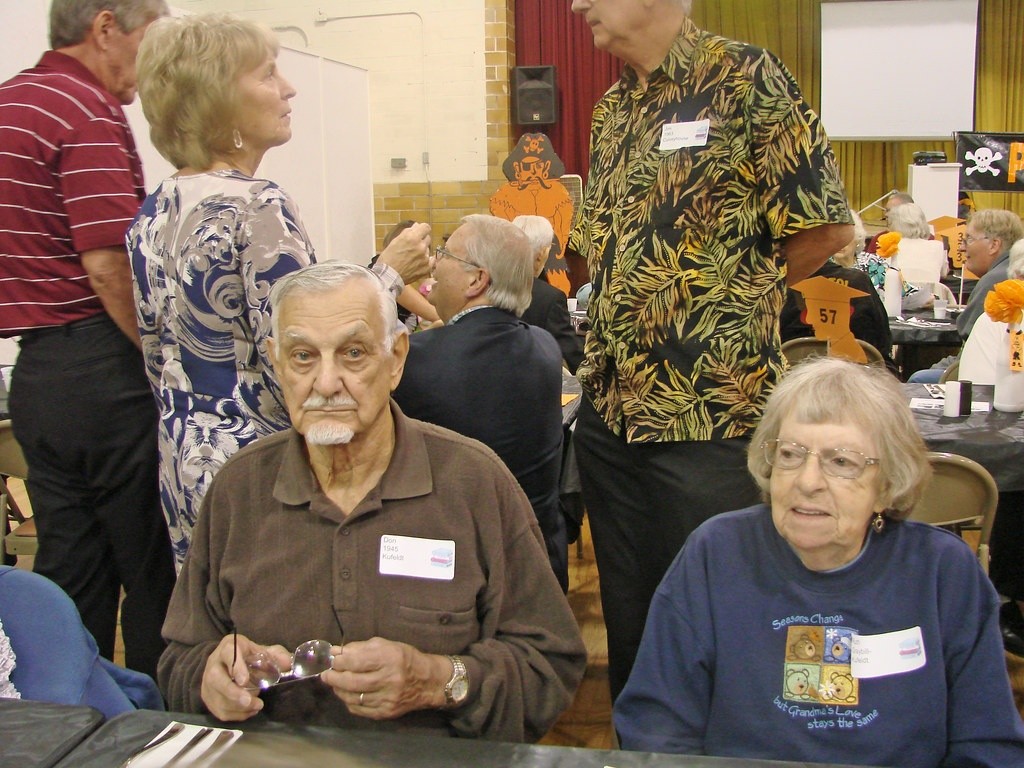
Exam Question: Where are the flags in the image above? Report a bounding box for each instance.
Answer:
[955,130,1024,192]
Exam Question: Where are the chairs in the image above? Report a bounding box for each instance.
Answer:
[906,452,998,577]
[0,418,39,568]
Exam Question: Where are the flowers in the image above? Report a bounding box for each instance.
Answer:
[875,232,902,258]
[984,279,1024,325]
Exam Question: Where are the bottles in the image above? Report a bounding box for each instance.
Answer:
[944,381,961,418]
[960,380,972,416]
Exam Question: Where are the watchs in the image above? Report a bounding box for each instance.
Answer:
[438,653,471,711]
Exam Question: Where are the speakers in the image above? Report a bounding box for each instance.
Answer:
[512,65,558,125]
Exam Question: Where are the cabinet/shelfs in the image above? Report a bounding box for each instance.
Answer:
[123,45,375,270]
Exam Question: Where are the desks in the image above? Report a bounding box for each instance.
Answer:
[55,709,870,768]
[0,697,105,768]
[900,383,1023,656]
[885,306,964,382]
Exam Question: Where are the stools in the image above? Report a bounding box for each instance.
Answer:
[781,337,885,368]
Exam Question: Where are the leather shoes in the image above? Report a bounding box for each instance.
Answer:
[999,601,1024,653]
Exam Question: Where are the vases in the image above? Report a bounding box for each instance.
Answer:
[884,255,901,318]
[993,322,1024,412]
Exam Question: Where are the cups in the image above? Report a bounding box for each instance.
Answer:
[933,299,947,319]
[567,299,577,317]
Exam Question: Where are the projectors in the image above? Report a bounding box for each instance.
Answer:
[914,150,947,163]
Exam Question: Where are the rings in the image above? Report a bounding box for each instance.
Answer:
[359,693,363,706]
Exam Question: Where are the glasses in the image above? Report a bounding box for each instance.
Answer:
[229,605,344,691]
[961,235,990,244]
[435,245,491,287]
[760,437,880,478]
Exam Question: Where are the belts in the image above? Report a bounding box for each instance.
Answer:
[22,311,111,337]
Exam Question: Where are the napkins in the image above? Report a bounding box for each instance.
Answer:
[126,720,244,768]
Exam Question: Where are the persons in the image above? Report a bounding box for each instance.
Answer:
[0,564,134,719]
[776,183,1024,657]
[609,349,1024,767]
[351,208,588,597]
[156,257,594,746]
[124,13,435,572]
[0,0,176,685]
[568,0,856,745]
